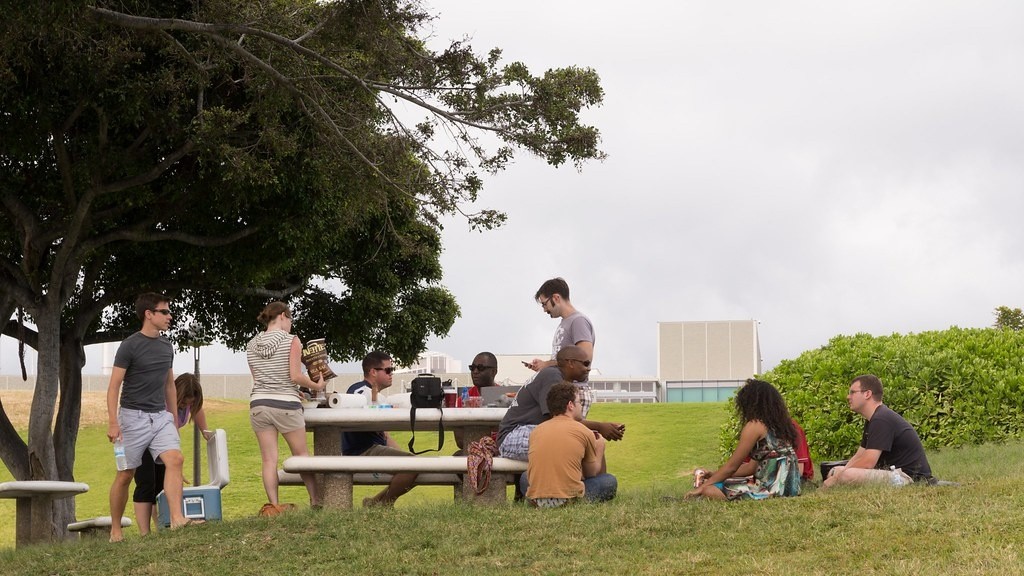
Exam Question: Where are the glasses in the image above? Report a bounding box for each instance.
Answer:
[150,309,174,315]
[565,359,591,367]
[542,295,560,308]
[468,365,496,372]
[290,317,293,323]
[848,389,873,396]
[368,366,396,374]
[573,399,586,406]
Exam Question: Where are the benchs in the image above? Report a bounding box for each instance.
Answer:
[276,455,529,512]
[67,515,132,542]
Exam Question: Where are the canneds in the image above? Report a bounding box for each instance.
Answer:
[693,469,706,488]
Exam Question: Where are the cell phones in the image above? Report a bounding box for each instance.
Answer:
[617,425,626,430]
[522,361,533,366]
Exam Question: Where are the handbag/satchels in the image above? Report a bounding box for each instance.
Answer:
[410,373,445,408]
[467,435,500,496]
[259,503,298,516]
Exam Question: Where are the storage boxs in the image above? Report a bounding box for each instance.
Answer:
[156,428,230,530]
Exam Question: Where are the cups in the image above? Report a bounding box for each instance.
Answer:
[444,393,457,407]
[592,430,599,440]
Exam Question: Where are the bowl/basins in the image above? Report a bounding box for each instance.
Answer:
[465,396,483,408]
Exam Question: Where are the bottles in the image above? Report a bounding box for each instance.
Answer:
[441,378,452,407]
[461,386,468,404]
[114,438,127,471]
[890,464,904,486]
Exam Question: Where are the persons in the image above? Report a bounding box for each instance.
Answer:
[524,277,595,418]
[683,379,815,502]
[103,292,207,542]
[496,345,625,463]
[132,372,214,537]
[246,301,330,517]
[823,374,939,491]
[453,352,514,480]
[519,381,618,508]
[340,351,422,508]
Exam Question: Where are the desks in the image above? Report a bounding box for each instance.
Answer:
[302,407,508,510]
[0,481,89,546]
